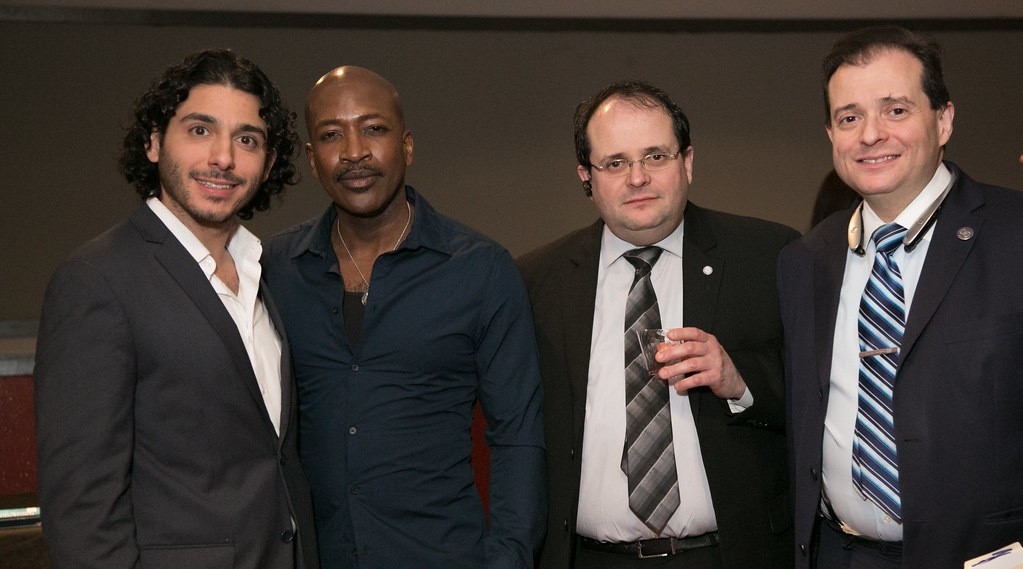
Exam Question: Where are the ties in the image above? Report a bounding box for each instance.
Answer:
[850,222,907,523]
[622,245,681,536]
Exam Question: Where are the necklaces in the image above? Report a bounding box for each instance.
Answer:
[337,202,412,305]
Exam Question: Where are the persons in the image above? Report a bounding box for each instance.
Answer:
[778,25,1023,569]
[259,66,552,569]
[32,49,318,569]
[515,77,805,569]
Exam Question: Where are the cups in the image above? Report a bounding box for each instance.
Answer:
[636,329,686,375]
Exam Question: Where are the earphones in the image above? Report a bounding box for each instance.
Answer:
[582,180,593,197]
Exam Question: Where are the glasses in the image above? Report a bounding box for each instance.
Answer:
[589,149,680,177]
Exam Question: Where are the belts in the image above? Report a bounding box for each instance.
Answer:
[577,532,722,558]
